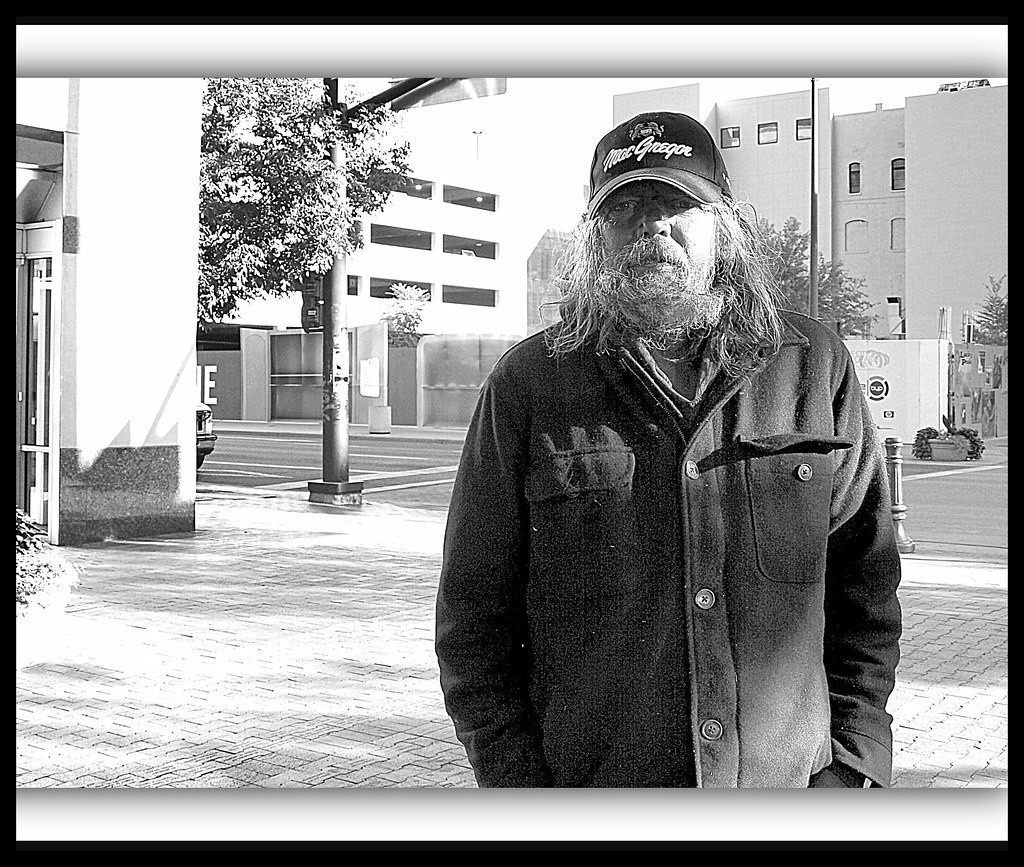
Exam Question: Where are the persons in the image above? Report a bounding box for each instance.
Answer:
[435,113,905,788]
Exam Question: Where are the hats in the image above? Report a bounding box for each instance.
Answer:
[585,110,731,220]
